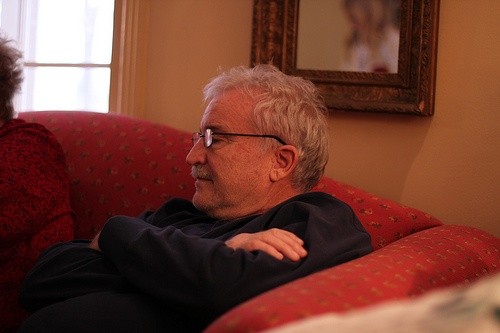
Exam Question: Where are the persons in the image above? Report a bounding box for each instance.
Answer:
[0,37,74,333]
[14,63,372,333]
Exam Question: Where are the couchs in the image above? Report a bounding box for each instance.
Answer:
[15,111,500,333]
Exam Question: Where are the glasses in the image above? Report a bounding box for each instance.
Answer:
[191,128,288,149]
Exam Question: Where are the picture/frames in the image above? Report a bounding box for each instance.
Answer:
[250,0,440,116]
